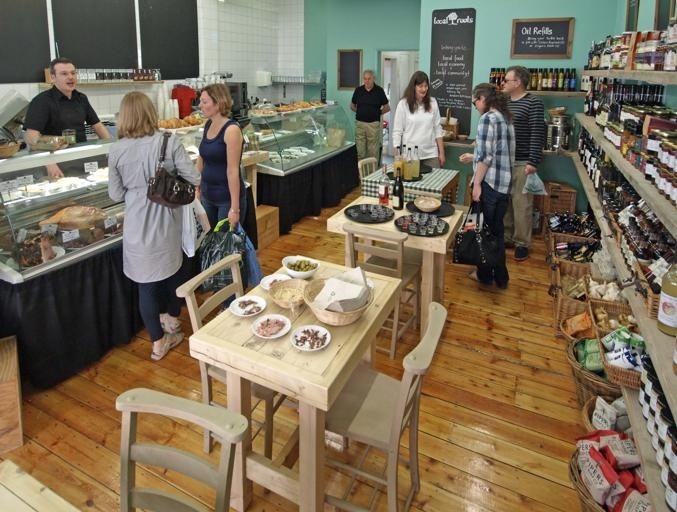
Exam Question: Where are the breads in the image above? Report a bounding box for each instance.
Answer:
[157,114,203,129]
[276,102,321,112]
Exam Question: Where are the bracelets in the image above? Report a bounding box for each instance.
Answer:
[230,208,240,214]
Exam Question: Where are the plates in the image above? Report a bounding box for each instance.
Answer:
[229,295,269,318]
[251,315,291,339]
[288,324,330,351]
[259,274,290,289]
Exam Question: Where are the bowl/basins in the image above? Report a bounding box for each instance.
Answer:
[281,256,319,280]
[414,196,440,213]
[0,144,23,159]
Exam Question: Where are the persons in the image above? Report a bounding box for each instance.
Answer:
[194,84,247,316]
[392,71,446,168]
[350,70,390,188]
[471,66,544,261]
[459,83,516,289]
[22,57,112,177]
[108,91,202,360]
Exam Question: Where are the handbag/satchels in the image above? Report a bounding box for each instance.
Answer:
[145,130,196,209]
[450,199,501,270]
[197,215,249,294]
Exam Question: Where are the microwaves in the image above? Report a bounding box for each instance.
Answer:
[193,80,249,120]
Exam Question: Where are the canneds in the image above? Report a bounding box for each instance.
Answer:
[604,103,677,207]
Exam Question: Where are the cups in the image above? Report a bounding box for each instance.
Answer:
[154,85,179,123]
[61,129,78,145]
[184,74,223,90]
[95,72,134,79]
[346,205,393,218]
[398,212,445,234]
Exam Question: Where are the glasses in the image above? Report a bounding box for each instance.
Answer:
[501,78,518,84]
[471,96,481,108]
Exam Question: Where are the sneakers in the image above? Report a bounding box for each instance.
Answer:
[511,246,529,261]
[157,312,183,335]
[149,330,185,361]
[502,241,514,249]
[463,266,509,289]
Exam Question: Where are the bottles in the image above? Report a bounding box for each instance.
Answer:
[378,164,389,206]
[392,166,405,208]
[133,67,161,81]
[488,66,578,91]
[570,123,677,512]
[465,219,474,228]
[583,77,666,119]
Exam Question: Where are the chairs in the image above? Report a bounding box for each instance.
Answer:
[171,252,286,494]
[340,221,423,361]
[358,156,376,179]
[320,302,447,512]
[104,385,247,511]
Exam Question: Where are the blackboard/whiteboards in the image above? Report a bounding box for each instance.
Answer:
[51,0,138,69]
[0,0,51,83]
[139,0,199,80]
[511,17,575,58]
[337,48,363,90]
[429,8,476,135]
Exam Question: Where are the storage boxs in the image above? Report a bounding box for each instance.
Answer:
[255,203,282,251]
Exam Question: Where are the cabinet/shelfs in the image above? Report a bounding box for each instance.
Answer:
[252,101,357,238]
[0,119,251,396]
[466,175,575,232]
[489,87,587,157]
[569,64,677,512]
